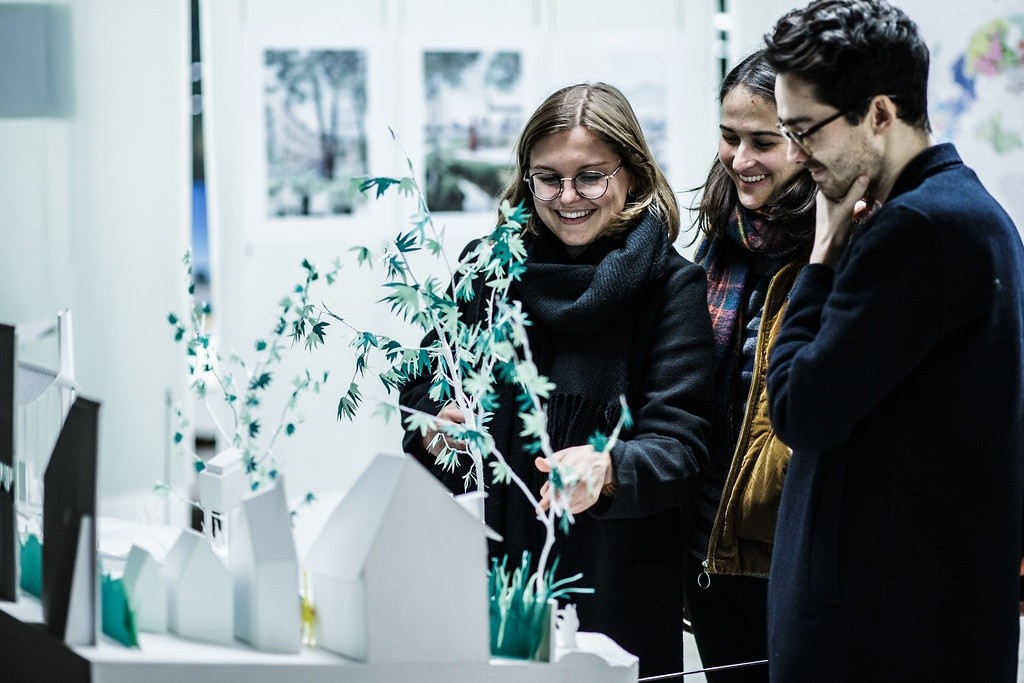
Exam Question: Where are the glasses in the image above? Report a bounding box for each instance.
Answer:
[776,95,898,157]
[522,163,624,200]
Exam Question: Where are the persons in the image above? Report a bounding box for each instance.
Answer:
[396,82,724,683]
[680,50,820,683]
[762,0,1024,683]
